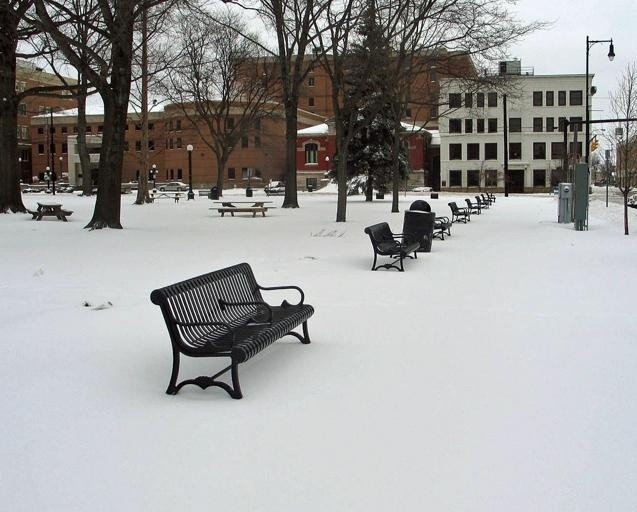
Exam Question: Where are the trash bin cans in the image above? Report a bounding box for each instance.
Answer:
[402,210,435,253]
[211,187,219,200]
[246,190,252,197]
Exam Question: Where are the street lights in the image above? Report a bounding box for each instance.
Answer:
[59,156,63,179]
[150,163,159,193]
[186,144,195,200]
[44,166,52,188]
[585,36,616,166]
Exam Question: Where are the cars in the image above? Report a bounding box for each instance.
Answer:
[129,180,139,190]
[264,180,285,193]
[157,181,190,192]
[22,186,75,195]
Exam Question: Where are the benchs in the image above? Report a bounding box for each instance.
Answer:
[448,190,496,224]
[150,197,186,203]
[365,222,421,271]
[26,208,74,222]
[209,207,277,217]
[151,262,314,399]
[433,216,452,240]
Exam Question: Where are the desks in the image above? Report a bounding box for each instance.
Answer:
[32,202,68,222]
[213,202,273,217]
[150,193,185,203]
